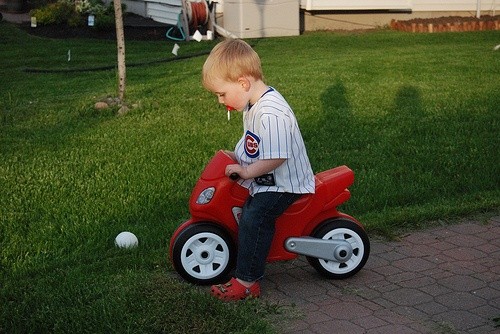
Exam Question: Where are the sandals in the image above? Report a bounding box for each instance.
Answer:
[210,276,261,302]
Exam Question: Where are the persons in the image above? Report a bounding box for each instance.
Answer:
[200,38,315,301]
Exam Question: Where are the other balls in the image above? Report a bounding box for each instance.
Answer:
[114,231,138,248]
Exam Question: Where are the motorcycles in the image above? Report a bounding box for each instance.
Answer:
[170,150,370,286]
